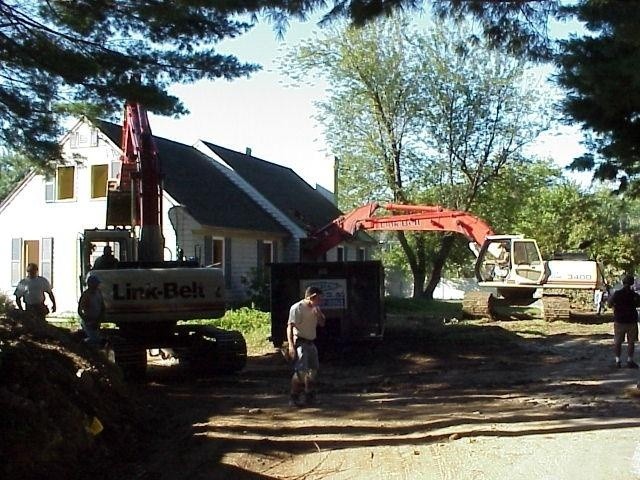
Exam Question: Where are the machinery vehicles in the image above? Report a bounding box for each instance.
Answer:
[77,73,249,383]
[309,200,609,322]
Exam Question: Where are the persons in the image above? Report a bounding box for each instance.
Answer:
[14,263,56,322]
[78,272,105,351]
[607,275,640,368]
[286,286,326,409]
[94,245,118,270]
[485,243,510,280]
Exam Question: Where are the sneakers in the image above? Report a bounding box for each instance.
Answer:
[615,362,620,368]
[626,361,638,368]
